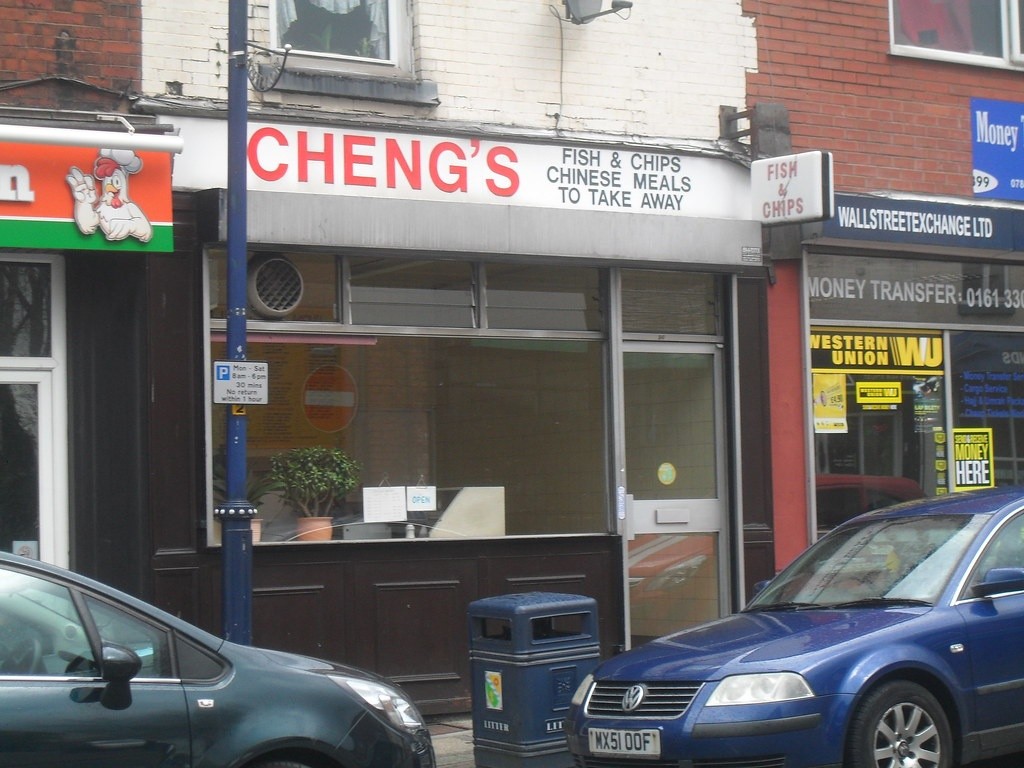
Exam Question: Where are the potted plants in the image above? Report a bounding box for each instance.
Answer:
[268,445,363,541]
[211,452,288,543]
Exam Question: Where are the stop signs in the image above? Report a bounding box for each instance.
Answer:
[302,364,359,433]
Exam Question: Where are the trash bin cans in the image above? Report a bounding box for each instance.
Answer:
[465,592,601,768]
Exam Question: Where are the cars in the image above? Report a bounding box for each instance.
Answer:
[564,486,1024,768]
[258,519,459,544]
[625,476,934,646]
[0,552,436,768]
